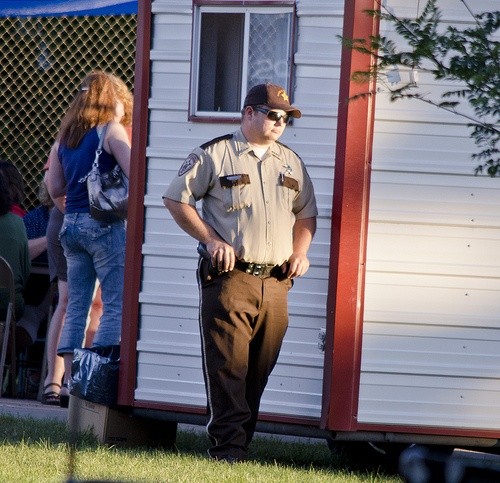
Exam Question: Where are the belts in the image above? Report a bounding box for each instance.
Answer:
[234,262,281,277]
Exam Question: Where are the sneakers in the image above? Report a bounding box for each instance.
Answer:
[77,121,131,223]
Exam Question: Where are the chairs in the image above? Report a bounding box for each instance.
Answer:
[0,255,19,398]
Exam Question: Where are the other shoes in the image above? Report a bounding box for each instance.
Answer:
[221,454,233,462]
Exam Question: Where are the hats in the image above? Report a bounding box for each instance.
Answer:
[244,84,301,118]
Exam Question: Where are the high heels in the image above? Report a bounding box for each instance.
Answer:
[59,383,70,408]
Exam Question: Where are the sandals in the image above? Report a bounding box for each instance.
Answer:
[41,383,61,405]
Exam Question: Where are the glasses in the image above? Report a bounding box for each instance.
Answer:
[254,107,294,126]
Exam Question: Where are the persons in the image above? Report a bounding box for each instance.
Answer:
[166,84,317,460]
[45,72,133,407]
[26,172,64,392]
[39,141,103,405]
[0,161,45,396]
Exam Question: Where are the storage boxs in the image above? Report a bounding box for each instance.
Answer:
[67,344,176,448]
